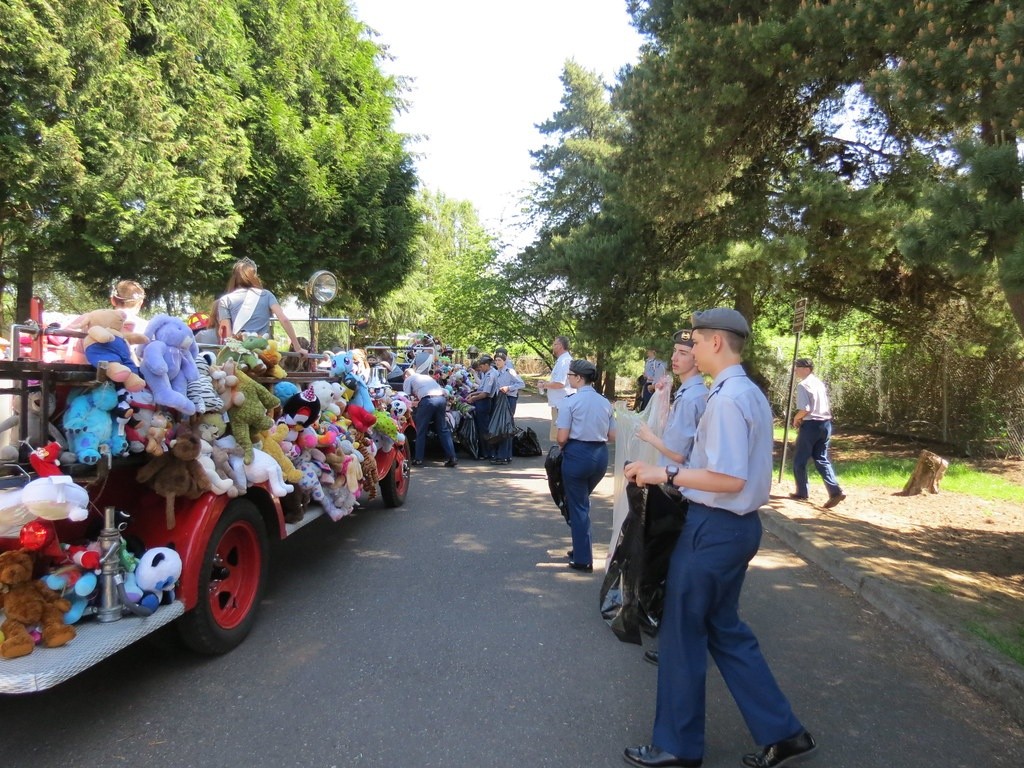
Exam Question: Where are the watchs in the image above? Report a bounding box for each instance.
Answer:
[666,464,679,485]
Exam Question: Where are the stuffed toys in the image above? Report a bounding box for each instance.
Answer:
[0,309,478,658]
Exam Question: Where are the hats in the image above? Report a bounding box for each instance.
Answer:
[795,357,814,367]
[672,328,694,347]
[690,307,750,339]
[471,348,508,369]
[569,359,596,376]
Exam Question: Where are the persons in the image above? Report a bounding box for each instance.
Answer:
[789,358,846,508]
[538,337,577,452]
[465,348,525,464]
[555,360,617,573]
[374,343,404,383]
[65,280,148,366]
[624,307,820,768]
[187,257,311,373]
[280,336,310,372]
[402,368,458,467]
[318,353,332,367]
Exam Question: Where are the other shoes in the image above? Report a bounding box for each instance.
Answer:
[643,650,659,665]
[823,492,847,508]
[790,492,807,500]
[490,457,512,465]
[411,460,423,467]
[445,457,458,466]
[421,395,445,401]
[566,550,593,573]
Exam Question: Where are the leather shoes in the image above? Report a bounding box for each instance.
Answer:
[623,745,704,768]
[740,728,817,768]
